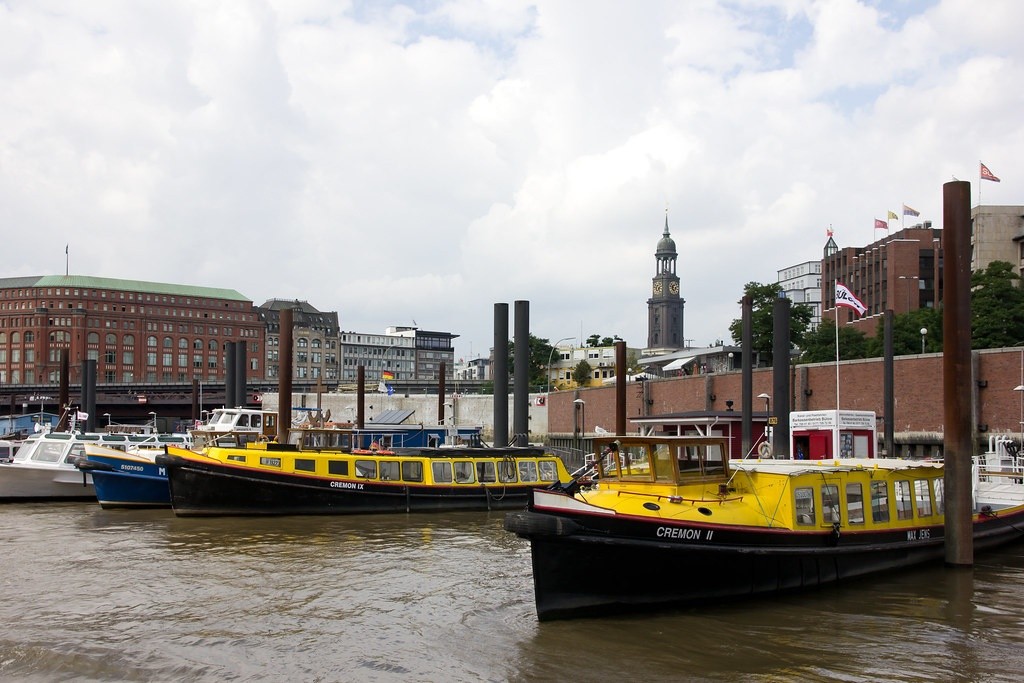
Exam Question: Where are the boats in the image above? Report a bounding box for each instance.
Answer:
[0,406,339,503]
[73,443,172,510]
[156,438,575,517]
[503,433,1024,623]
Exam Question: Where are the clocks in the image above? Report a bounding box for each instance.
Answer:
[669,281,679,295]
[653,281,662,295]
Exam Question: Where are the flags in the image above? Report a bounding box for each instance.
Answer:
[388,384,395,395]
[904,205,920,217]
[875,220,887,229]
[889,211,898,219]
[378,382,387,392]
[836,283,868,317]
[981,163,1000,182]
[382,371,394,380]
[827,226,834,236]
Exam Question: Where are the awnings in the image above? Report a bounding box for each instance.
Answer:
[663,357,695,371]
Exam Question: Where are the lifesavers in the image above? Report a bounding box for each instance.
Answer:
[258,434,270,442]
[758,441,773,458]
[351,448,373,455]
[376,450,396,456]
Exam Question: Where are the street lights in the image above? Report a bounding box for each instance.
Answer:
[148,412,157,433]
[757,393,771,443]
[380,343,403,394]
[1013,386,1024,473]
[200,410,209,424]
[345,407,355,424]
[728,353,733,370]
[548,337,575,392]
[628,368,632,381]
[103,413,111,436]
[921,328,927,354]
[356,351,371,382]
[899,276,919,324]
